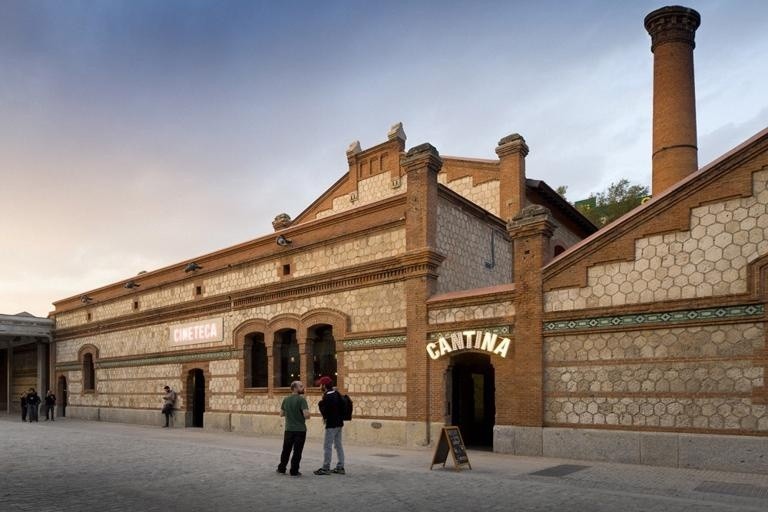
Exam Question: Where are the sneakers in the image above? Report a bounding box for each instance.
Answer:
[330,467,345,475]
[44,417,49,421]
[51,417,55,421]
[312,468,331,475]
[162,425,168,428]
[297,472,301,475]
[276,469,285,475]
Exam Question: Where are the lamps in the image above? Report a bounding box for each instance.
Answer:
[124,282,140,289]
[80,294,92,303]
[276,235,292,245]
[184,261,202,273]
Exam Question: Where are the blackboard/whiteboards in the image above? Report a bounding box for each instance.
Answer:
[443,426,472,467]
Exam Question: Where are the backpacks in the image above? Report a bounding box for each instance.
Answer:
[333,392,353,421]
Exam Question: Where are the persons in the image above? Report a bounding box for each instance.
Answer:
[32,391,41,420]
[27,387,38,423]
[314,376,353,477]
[19,390,29,422]
[273,380,312,478]
[161,385,176,428]
[44,389,57,421]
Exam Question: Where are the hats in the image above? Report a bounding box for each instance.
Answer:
[314,376,333,386]
